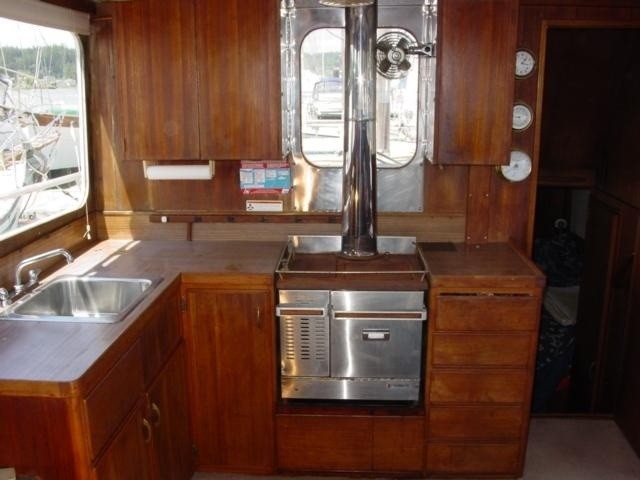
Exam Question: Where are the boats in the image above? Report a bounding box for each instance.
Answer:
[0,35,82,237]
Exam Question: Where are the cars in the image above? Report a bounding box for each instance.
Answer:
[306,77,343,119]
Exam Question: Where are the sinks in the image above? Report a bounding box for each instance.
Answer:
[0,275,164,325]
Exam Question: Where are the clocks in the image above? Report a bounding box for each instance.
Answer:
[513,100,534,133]
[515,48,539,79]
[496,148,532,183]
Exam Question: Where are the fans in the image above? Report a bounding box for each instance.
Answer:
[377,32,432,81]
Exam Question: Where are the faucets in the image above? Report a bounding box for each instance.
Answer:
[13,248,74,290]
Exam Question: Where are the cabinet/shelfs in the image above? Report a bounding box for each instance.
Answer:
[427,276,547,478]
[180,274,278,480]
[113,0,292,162]
[0,287,194,480]
[423,0,519,165]
